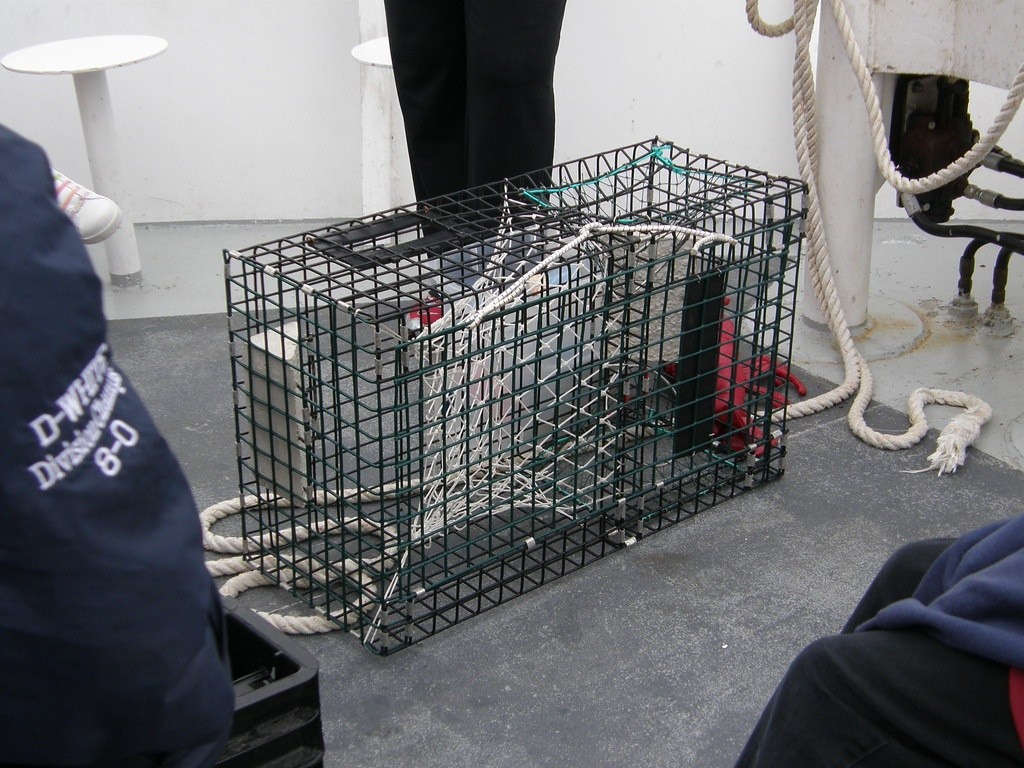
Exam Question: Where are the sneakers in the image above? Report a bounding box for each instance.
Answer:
[48,168,124,243]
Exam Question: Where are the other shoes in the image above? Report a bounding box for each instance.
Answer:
[406,287,446,337]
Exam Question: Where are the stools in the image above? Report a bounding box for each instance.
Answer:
[0,34,170,290]
[349,35,397,249]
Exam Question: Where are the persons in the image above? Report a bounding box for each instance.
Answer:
[384,0,568,260]
[0,123,235,767]
[735,515,1024,768]
[48,168,124,245]
